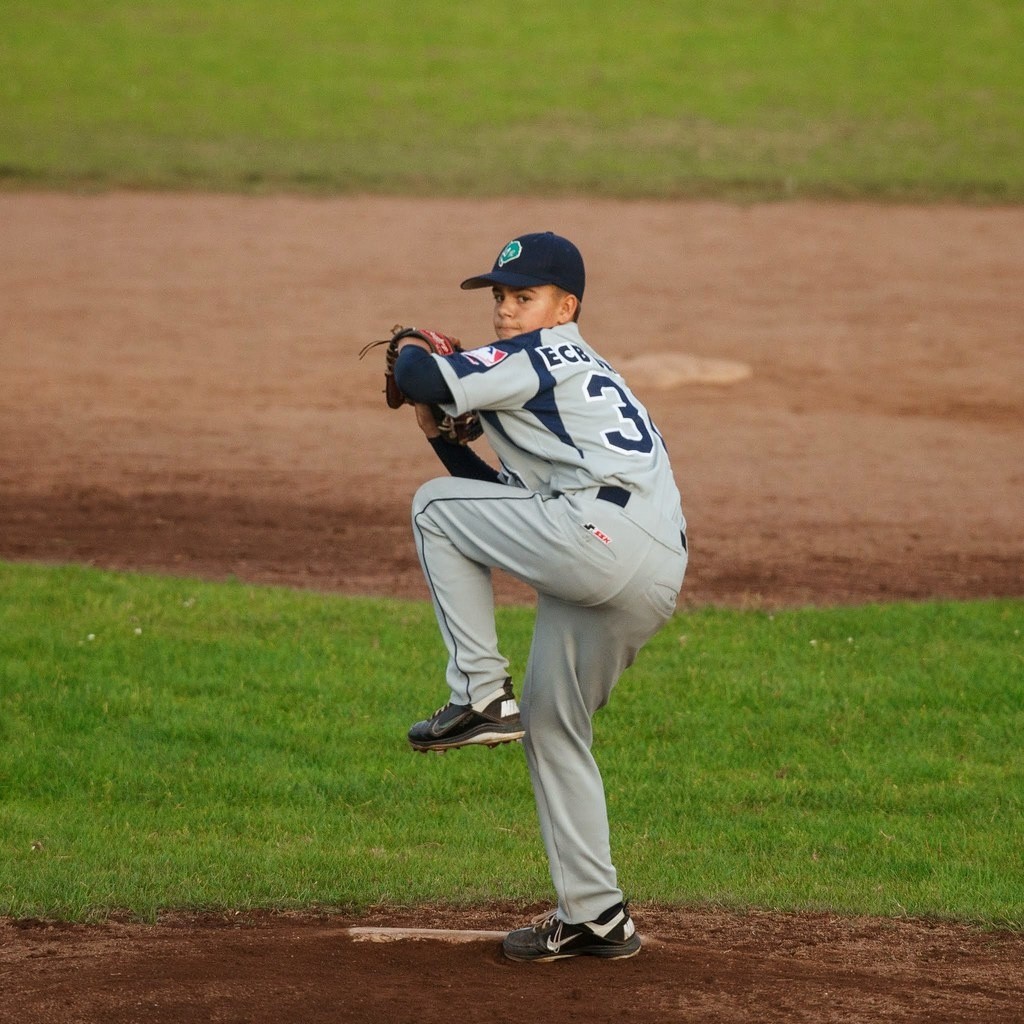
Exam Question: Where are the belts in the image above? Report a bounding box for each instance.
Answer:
[597,486,687,551]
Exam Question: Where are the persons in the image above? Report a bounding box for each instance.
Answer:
[357,231,690,962]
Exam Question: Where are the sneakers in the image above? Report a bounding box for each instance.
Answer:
[503,898,642,962]
[409,676,526,755]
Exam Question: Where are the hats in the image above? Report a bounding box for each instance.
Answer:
[461,231,586,302]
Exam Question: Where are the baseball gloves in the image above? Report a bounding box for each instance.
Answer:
[382,325,480,443]
